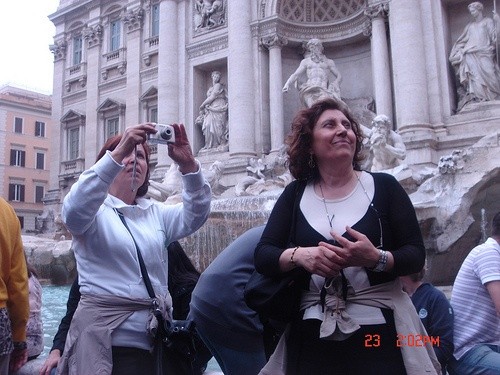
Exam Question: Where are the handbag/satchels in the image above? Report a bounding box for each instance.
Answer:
[149,329,207,375]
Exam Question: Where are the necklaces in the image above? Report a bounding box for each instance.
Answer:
[317,173,383,248]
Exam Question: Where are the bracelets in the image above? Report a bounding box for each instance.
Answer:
[290,245,299,267]
[372,249,388,272]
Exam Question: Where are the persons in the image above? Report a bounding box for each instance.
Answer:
[448,0,500,111]
[54,121,212,375]
[282,38,343,106]
[195,71,229,148]
[0,197,454,375]
[358,115,406,172]
[255,96,427,374]
[196,0,226,27]
[450,213,500,375]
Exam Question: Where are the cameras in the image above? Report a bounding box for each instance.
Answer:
[148,123,176,144]
